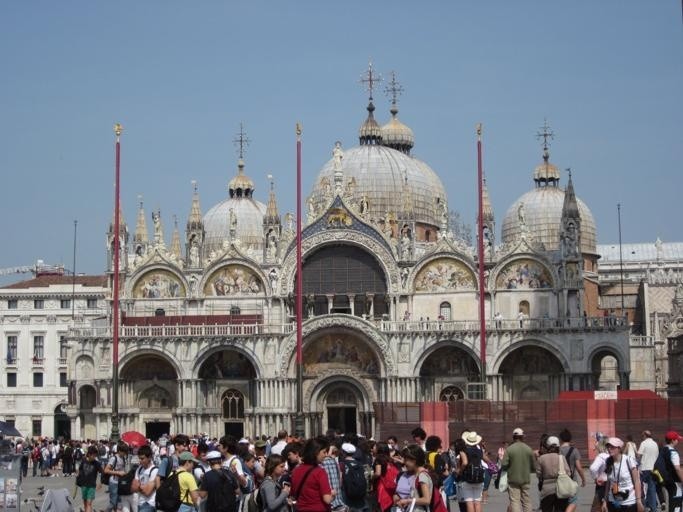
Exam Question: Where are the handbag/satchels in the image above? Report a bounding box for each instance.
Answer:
[556,473,578,499]
[463,463,484,483]
[611,488,629,501]
[102,455,116,485]
[499,470,508,492]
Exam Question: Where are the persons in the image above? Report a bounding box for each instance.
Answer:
[583,306,628,327]
[403,310,410,325]
[437,313,444,329]
[493,314,498,328]
[496,312,502,328]
[517,310,523,327]
[0,427,682,512]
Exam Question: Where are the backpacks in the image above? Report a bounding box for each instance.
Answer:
[241,476,272,512]
[343,461,367,508]
[416,470,449,512]
[155,471,189,512]
[377,454,400,489]
[653,448,677,483]
[118,464,139,494]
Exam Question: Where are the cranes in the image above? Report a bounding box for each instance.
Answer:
[0,260,66,278]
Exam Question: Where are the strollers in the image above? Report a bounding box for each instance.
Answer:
[24,485,79,512]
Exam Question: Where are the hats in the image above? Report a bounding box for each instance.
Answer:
[666,432,683,440]
[513,427,525,436]
[205,451,222,460]
[547,436,560,448]
[255,440,266,448]
[605,438,624,448]
[342,443,356,453]
[462,431,482,445]
[179,452,199,464]
